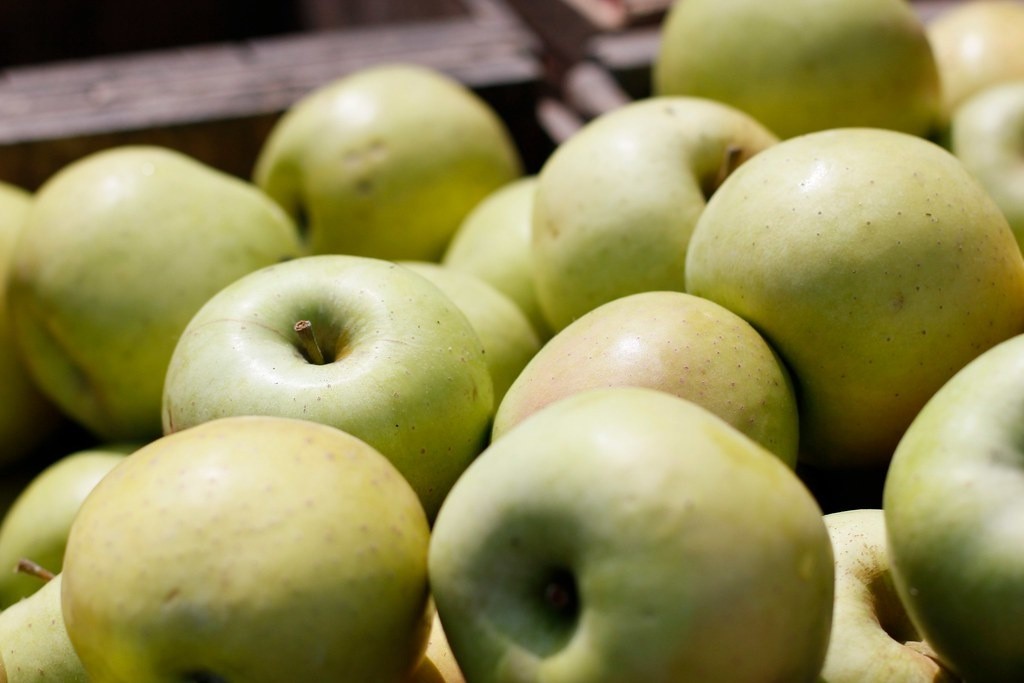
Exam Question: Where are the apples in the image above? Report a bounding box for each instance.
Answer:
[0,0,1024,683]
